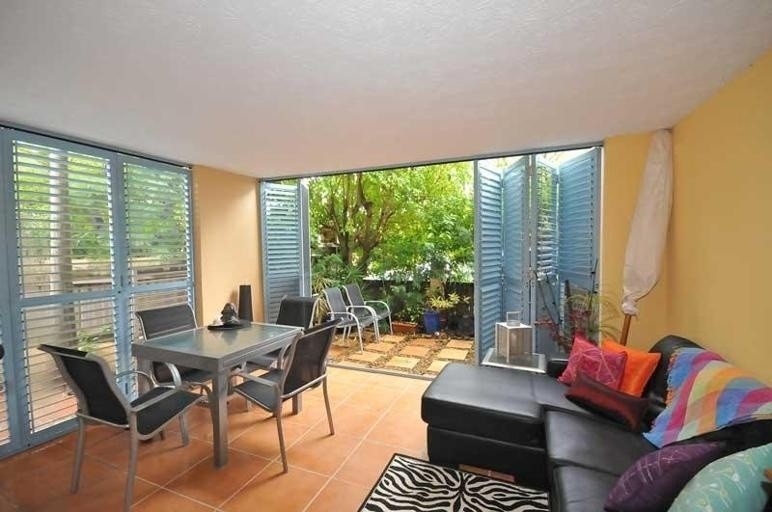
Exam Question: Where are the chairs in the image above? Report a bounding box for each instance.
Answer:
[36,343,212,512]
[342,281,393,339]
[134,301,213,448]
[242,294,321,415]
[228,317,343,476]
[322,287,379,355]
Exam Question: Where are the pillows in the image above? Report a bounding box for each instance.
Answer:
[564,370,651,433]
[667,441,772,512]
[599,335,662,398]
[556,334,627,391]
[603,442,728,512]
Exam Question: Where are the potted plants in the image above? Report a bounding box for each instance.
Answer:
[392,302,425,337]
[421,286,445,334]
[430,292,461,332]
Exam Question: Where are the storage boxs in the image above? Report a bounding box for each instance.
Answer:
[493,319,535,364]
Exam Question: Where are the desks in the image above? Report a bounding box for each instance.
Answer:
[480,346,547,375]
[131,322,306,467]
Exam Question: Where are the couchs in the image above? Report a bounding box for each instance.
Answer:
[420,334,772,512]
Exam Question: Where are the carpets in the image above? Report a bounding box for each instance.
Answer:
[357,452,551,512]
